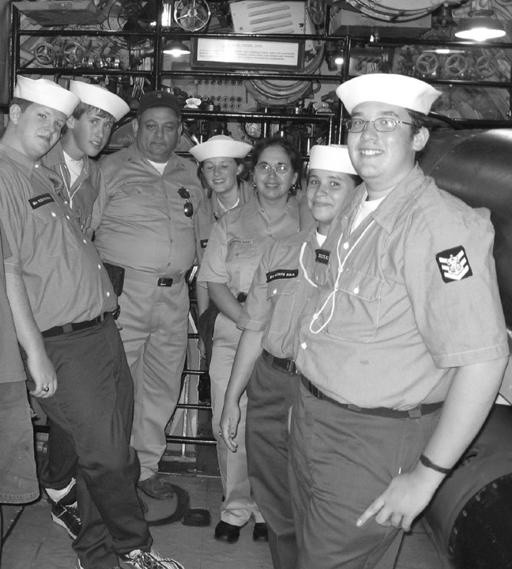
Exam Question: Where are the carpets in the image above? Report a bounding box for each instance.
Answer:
[156,410,230,480]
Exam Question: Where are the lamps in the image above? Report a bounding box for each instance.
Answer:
[455,10,505,46]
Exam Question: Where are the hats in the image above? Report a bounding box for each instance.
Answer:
[189,135,252,162]
[336,73,442,115]
[14,75,130,123]
[309,145,357,175]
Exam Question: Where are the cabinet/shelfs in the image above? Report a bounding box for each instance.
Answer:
[0,0,158,114]
[159,31,348,163]
[348,34,511,145]
[161,295,217,448]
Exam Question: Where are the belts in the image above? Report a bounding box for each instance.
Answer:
[263,350,290,369]
[300,375,443,417]
[42,313,106,337]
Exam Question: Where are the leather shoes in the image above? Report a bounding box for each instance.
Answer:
[139,476,171,499]
[253,523,268,541]
[216,521,239,542]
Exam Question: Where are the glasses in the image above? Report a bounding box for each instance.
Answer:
[346,118,414,133]
[177,188,193,217]
[258,164,289,174]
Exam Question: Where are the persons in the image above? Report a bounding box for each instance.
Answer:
[2,72,511,567]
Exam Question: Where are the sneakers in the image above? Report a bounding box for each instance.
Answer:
[124,549,184,569]
[51,505,81,540]
